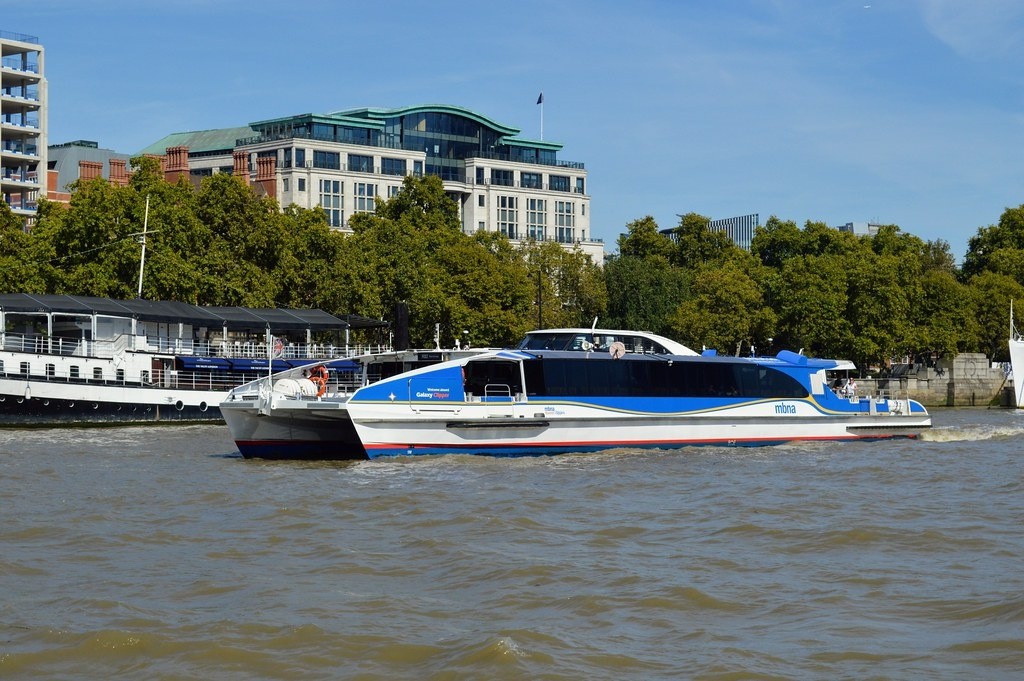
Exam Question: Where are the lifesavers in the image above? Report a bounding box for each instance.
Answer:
[310,376,325,396]
[311,366,328,383]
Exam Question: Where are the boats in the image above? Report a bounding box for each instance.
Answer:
[1008,300,1024,408]
[220,316,933,461]
[0,192,396,428]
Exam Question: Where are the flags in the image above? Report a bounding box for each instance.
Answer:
[537,93,543,104]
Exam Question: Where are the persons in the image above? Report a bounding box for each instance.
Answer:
[833,373,858,399]
